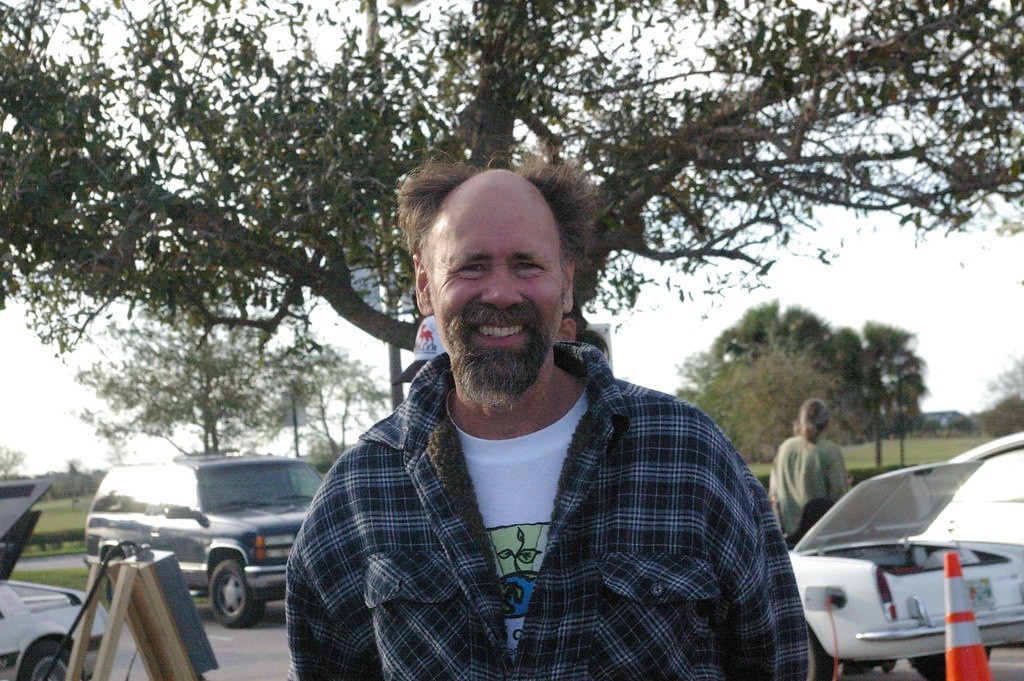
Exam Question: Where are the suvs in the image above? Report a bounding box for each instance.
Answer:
[83,450,326,633]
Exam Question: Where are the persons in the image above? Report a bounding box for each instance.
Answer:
[285,153,810,681]
[768,399,853,539]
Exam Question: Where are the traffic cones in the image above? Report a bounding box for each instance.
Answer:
[944,551,997,681]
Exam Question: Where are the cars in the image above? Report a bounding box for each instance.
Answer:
[0,479,118,681]
[783,459,1024,680]
[900,431,1024,549]
